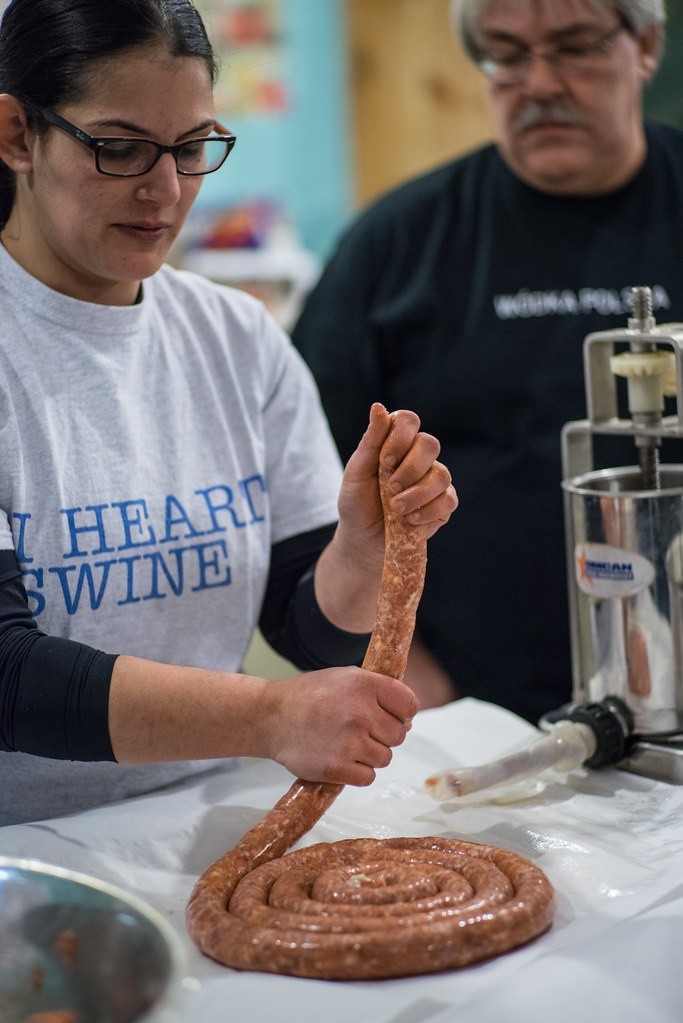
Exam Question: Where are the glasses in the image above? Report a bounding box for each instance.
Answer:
[461,13,624,88]
[11,87,239,182]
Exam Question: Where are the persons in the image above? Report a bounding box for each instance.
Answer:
[0,1,459,825]
[291,2,682,712]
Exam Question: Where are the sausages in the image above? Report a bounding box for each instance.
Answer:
[187,452,557,981]
